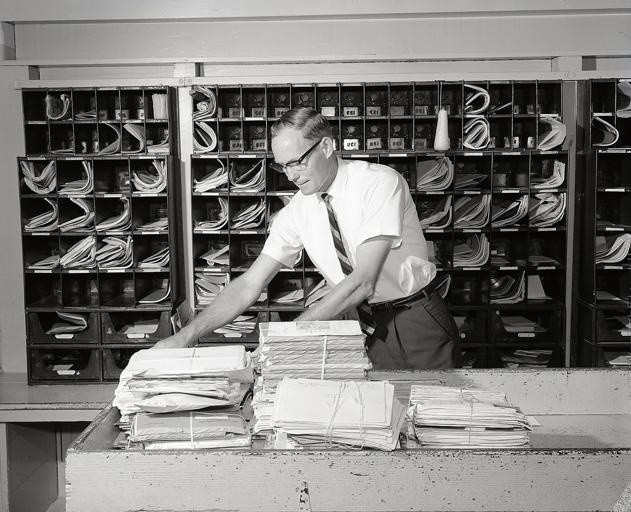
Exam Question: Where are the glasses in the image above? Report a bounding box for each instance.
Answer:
[270,140,322,173]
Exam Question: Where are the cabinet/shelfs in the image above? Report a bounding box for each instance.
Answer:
[16,77,629,389]
[64,368,628,512]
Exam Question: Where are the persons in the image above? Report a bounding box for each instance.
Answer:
[150,107,461,370]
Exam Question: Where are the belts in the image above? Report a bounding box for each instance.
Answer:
[373,279,437,311]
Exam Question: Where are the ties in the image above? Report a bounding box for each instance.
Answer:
[322,193,376,336]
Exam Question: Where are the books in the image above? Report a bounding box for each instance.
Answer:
[126,346,252,384]
[618,316,630,337]
[464,117,490,149]
[193,122,218,153]
[59,198,94,232]
[535,160,565,188]
[251,377,275,436]
[60,236,97,267]
[528,275,550,299]
[129,378,230,400]
[269,196,293,228]
[596,233,631,262]
[229,160,266,191]
[190,85,217,120]
[112,366,139,449]
[405,406,532,429]
[74,111,96,120]
[232,198,266,228]
[138,276,170,303]
[617,82,631,118]
[593,117,619,147]
[417,157,453,190]
[596,291,620,300]
[52,365,76,375]
[434,275,451,299]
[414,426,529,450]
[606,351,631,365]
[130,412,251,451]
[58,161,93,195]
[408,385,508,406]
[537,117,566,150]
[122,124,145,153]
[124,320,158,334]
[137,218,168,230]
[454,194,491,228]
[25,198,57,231]
[147,135,169,152]
[453,233,489,266]
[271,289,304,302]
[419,195,452,229]
[213,315,256,337]
[53,149,73,153]
[502,316,536,332]
[490,270,525,304]
[138,246,170,267]
[153,94,168,120]
[99,124,121,153]
[46,94,70,119]
[46,310,87,334]
[132,160,167,193]
[28,255,58,269]
[529,192,566,227]
[96,197,132,230]
[492,195,528,226]
[502,350,552,367]
[271,378,405,451]
[490,101,512,114]
[259,320,372,394]
[20,160,56,194]
[200,245,229,266]
[194,159,227,192]
[195,273,227,304]
[464,84,490,114]
[194,197,230,231]
